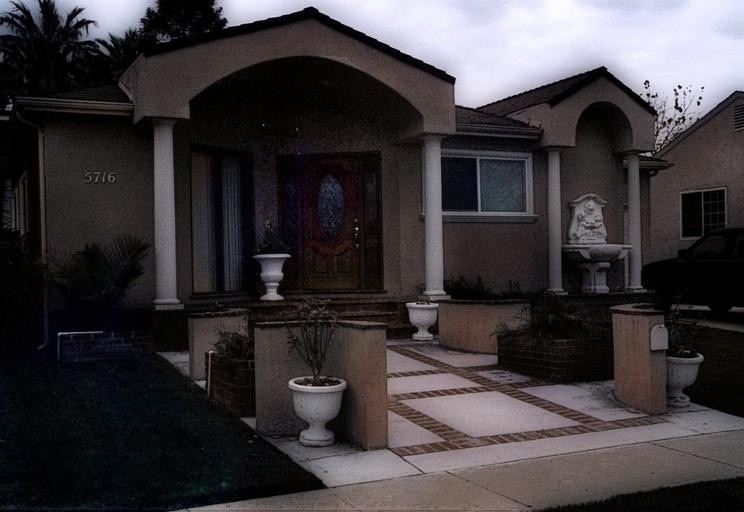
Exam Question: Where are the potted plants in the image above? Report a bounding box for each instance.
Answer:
[253,223,294,302]
[405,297,440,342]
[280,296,347,446]
[667,292,705,410]
